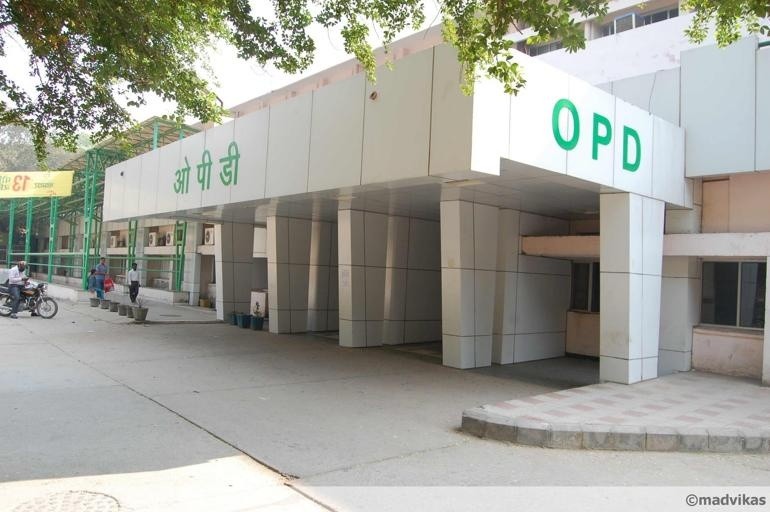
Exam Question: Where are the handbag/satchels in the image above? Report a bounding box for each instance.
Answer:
[104,278,115,293]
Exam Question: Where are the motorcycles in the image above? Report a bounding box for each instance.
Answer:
[0,278,58,318]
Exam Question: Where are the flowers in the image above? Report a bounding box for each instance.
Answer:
[252,302,262,317]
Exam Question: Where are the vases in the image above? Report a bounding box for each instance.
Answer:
[236,314,252,328]
[250,317,264,330]
[116,304,129,315]
[99,300,112,309]
[125,306,134,317]
[90,298,100,307]
[230,313,237,324]
[132,307,148,321]
[110,302,120,312]
[200,299,209,307]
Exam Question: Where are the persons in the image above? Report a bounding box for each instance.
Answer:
[128,263,140,303]
[9,261,39,318]
[86,268,105,305]
[96,257,109,297]
[103,274,115,292]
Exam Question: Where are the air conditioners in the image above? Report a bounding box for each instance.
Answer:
[204,228,214,244]
[148,232,158,247]
[166,232,174,245]
[109,236,118,248]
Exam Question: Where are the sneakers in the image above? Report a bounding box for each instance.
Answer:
[31,313,38,316]
[11,314,17,319]
